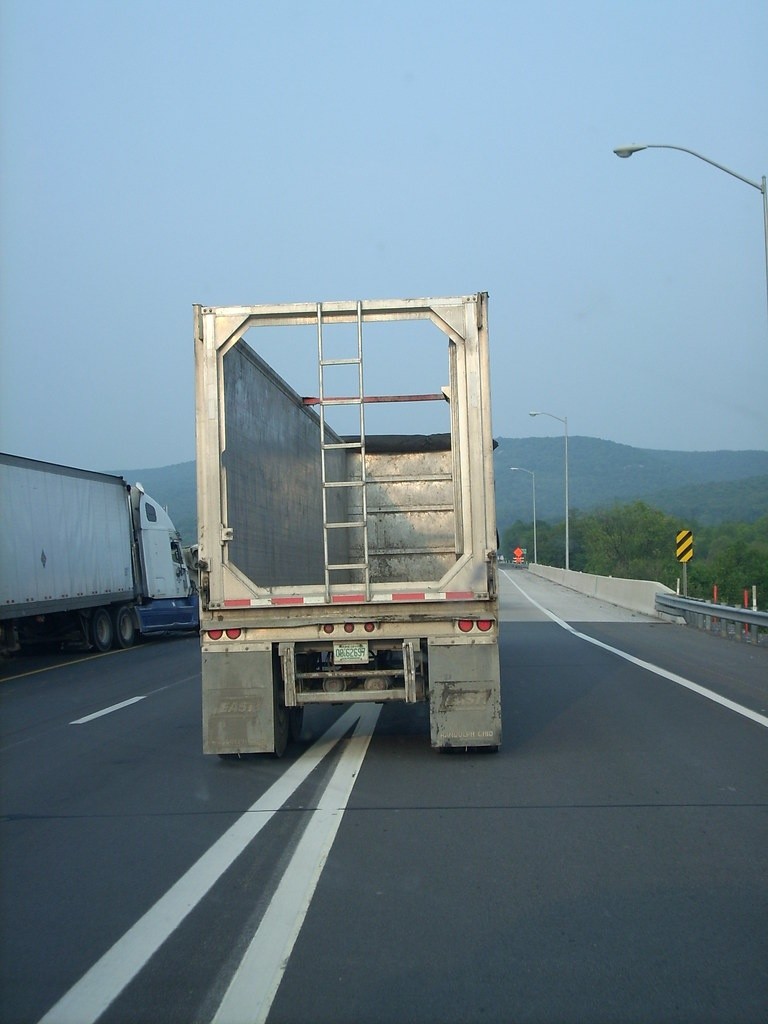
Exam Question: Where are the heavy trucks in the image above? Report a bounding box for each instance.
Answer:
[0,453,194,662]
[192,291,502,760]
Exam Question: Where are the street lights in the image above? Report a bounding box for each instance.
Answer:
[529,411,569,569]
[510,467,537,564]
[613,144,768,298]
